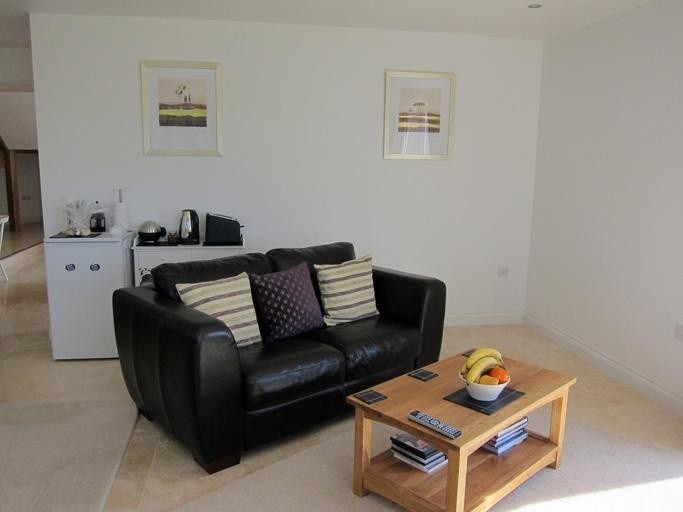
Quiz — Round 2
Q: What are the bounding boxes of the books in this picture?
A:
[480,416,529,456]
[389,429,449,474]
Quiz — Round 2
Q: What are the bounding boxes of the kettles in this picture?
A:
[179,210,200,244]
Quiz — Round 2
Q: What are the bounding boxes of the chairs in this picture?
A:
[0,215,10,281]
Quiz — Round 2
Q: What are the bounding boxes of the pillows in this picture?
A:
[250,262,324,342]
[314,254,380,326]
[176,272,262,348]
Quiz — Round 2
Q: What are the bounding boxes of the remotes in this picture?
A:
[408,410,462,439]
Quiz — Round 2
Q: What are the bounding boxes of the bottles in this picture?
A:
[90,213,105,232]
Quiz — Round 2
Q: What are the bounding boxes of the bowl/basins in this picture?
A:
[458,371,511,402]
[138,232,161,244]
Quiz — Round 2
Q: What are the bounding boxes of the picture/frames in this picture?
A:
[140,59,224,156]
[383,70,457,160]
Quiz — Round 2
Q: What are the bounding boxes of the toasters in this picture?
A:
[205,212,243,243]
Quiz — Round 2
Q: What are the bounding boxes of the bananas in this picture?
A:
[461,347,506,386]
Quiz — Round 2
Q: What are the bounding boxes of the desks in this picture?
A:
[346,347,577,512]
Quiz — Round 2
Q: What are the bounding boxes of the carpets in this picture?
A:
[0,399,139,512]
[168,409,683,512]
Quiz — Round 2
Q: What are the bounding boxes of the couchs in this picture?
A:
[113,242,447,474]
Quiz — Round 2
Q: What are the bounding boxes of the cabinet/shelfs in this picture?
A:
[43,232,136,360]
[131,235,247,287]
